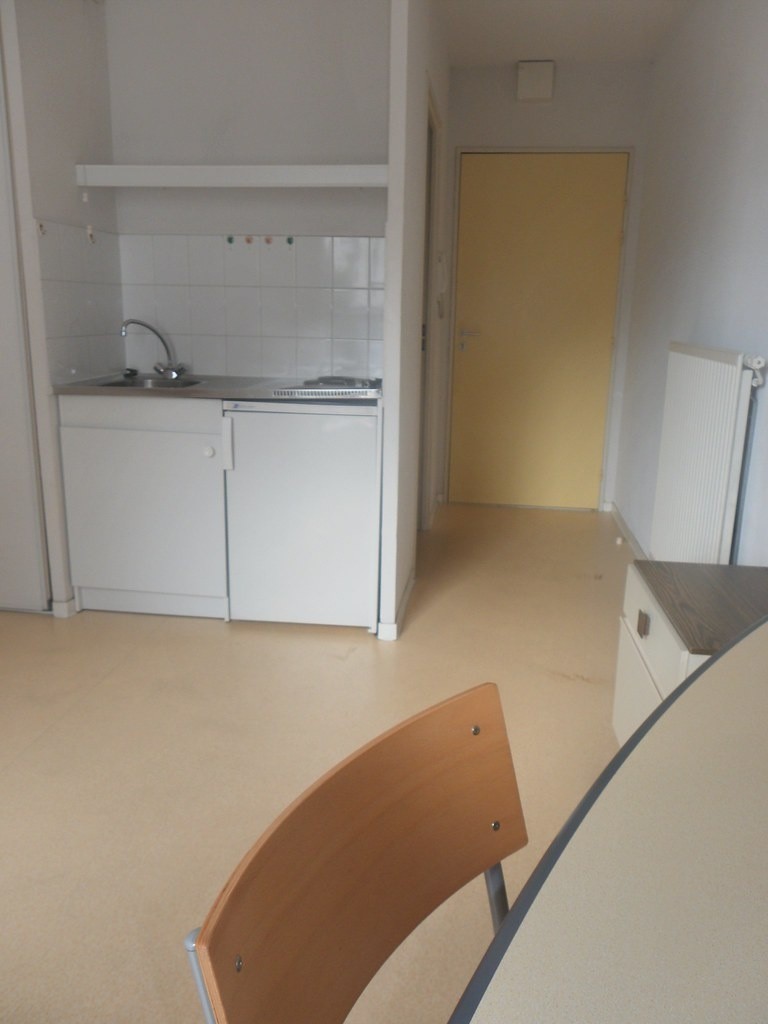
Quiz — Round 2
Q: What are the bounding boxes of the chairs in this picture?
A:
[189,676,528,1024]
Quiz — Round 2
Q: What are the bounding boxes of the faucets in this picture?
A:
[120,318,190,380]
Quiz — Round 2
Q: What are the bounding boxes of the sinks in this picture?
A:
[96,375,207,391]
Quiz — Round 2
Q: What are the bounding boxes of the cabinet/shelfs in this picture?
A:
[59,404,394,636]
[606,557,768,752]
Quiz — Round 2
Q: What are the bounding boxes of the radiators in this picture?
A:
[642,341,768,569]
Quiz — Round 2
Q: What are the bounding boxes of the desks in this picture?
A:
[447,610,768,1024]
